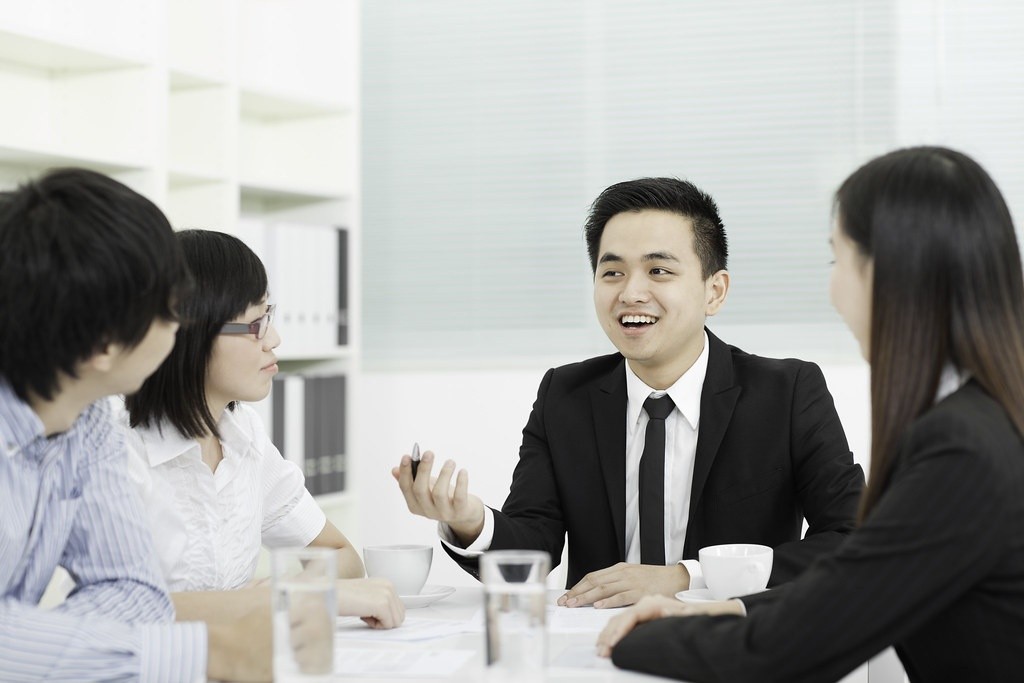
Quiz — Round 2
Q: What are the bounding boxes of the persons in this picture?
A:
[126,226,408,630]
[393,180,866,608]
[597,146,1024,683]
[0,167,273,683]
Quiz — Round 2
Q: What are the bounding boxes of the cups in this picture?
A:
[479,550,550,678]
[270,547,338,682]
[698,544,774,599]
[362,544,433,595]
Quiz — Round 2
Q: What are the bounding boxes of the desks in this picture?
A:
[274,568,874,683]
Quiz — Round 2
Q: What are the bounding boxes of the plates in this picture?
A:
[338,579,455,609]
[675,589,773,607]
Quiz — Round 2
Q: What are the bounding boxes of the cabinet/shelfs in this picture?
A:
[0,41,377,509]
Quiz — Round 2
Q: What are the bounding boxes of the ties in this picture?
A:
[639,394,676,566]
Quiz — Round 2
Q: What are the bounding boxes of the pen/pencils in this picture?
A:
[411,442,421,481]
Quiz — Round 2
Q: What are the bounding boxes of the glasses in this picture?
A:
[219,305,276,339]
